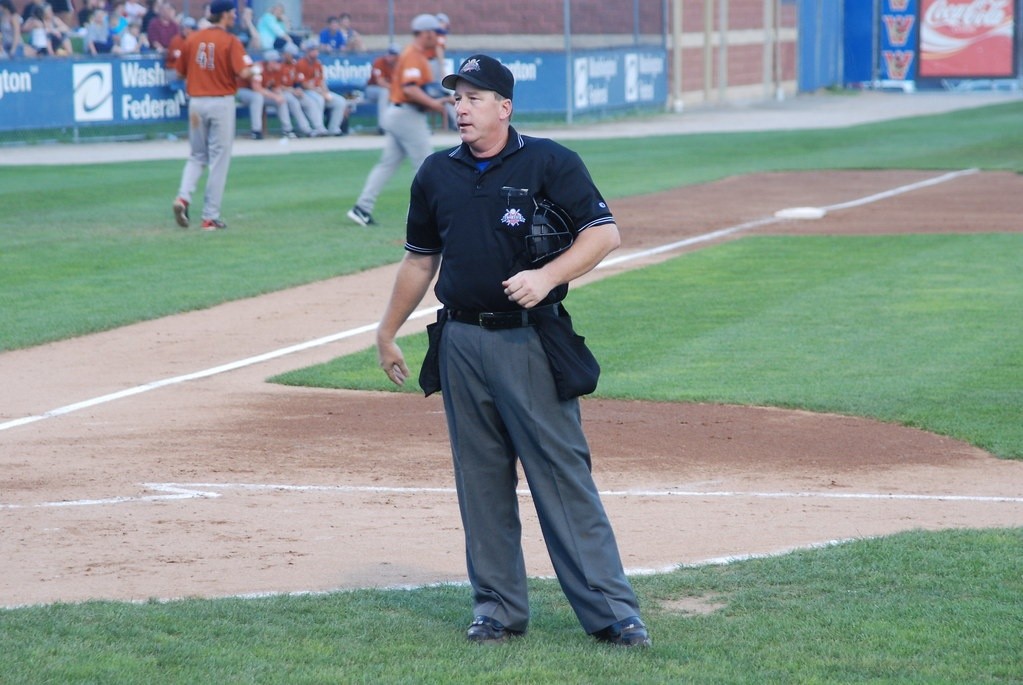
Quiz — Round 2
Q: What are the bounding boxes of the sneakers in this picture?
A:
[201,219,227,231]
[172,197,191,227]
[591,616,653,648]
[467,615,528,643]
[346,204,377,227]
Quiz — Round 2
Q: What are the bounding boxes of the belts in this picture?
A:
[445,308,538,329]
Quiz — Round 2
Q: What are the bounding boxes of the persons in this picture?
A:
[171,0,255,229]
[0,0,451,139]
[347,13,455,228]
[376,55,651,646]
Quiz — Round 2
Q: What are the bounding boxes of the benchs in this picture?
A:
[262,97,447,137]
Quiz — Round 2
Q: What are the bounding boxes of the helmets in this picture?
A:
[494,186,579,304]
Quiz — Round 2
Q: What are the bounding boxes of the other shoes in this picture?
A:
[282,131,297,139]
[250,132,263,139]
[307,128,341,137]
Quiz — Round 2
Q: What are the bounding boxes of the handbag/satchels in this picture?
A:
[528,302,601,402]
[418,310,448,398]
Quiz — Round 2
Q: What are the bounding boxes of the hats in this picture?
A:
[411,13,449,35]
[436,13,450,23]
[210,0,238,13]
[441,55,514,99]
